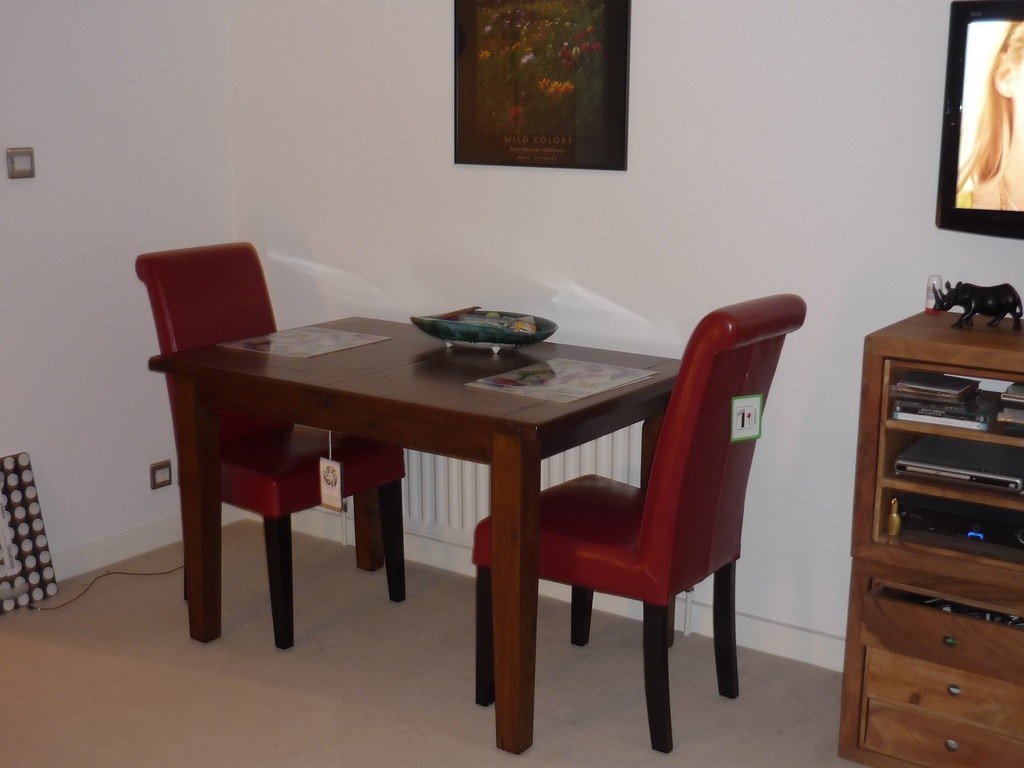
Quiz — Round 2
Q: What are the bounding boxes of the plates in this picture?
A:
[410,311,559,344]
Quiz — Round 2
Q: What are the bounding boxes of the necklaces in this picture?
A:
[1000,179,1006,211]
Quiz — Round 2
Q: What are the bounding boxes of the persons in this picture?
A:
[956,21,1024,211]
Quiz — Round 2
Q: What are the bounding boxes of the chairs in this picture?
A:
[473,292,807,754]
[136,240,408,650]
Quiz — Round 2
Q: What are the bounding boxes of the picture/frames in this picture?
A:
[452,0,630,171]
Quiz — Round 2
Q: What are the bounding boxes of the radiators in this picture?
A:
[345,417,645,550]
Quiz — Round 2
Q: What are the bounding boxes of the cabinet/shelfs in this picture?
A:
[837,309,1024,768]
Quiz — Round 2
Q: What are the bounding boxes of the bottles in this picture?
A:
[887,498,901,536]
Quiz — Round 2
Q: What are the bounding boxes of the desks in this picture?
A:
[148,318,686,705]
